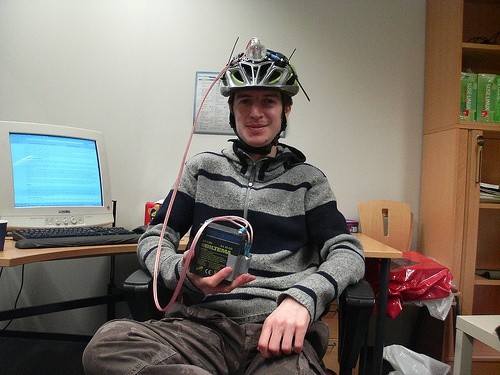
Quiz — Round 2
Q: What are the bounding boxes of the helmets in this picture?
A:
[220,49,299,97]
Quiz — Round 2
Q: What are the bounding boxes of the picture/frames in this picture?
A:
[192,71,285,137]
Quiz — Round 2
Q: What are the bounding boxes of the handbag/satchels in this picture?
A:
[383,345,451,375]
[376,251,454,301]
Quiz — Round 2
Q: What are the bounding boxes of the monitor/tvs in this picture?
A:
[0,121,114,230]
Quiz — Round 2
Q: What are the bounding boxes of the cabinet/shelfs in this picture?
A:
[417,0,500,375]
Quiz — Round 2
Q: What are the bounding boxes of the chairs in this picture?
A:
[123,268,376,375]
[357,200,455,366]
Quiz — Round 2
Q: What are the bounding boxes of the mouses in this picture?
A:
[132,225,146,234]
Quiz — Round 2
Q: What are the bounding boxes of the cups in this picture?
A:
[0,219,8,251]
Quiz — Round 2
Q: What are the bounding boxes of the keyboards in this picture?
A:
[12,227,128,241]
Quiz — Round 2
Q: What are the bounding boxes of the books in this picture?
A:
[480,182,500,203]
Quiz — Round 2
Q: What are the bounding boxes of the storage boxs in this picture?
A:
[476,73,500,122]
[459,71,477,121]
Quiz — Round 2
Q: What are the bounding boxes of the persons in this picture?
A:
[83,37,365,375]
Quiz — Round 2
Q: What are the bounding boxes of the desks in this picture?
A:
[0,233,404,375]
[453,314,500,375]
